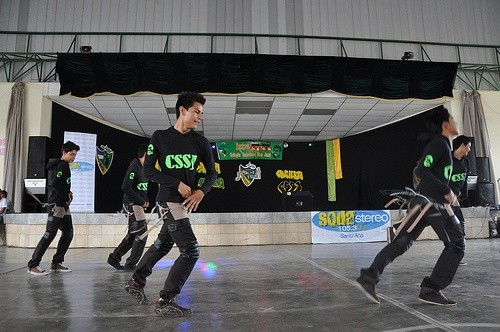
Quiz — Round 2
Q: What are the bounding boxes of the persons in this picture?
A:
[388,135,472,266]
[124,92,217,317]
[107,144,150,271]
[27,141,81,276]
[355,107,467,306]
[0,189,15,247]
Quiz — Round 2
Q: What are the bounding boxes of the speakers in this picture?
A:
[478,182,494,206]
[476,157,491,183]
[27,136,58,179]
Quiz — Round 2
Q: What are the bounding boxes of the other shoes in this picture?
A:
[125,267,136,272]
[107,259,125,270]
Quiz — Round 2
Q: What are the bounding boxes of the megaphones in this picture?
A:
[80,46,92,53]
[401,52,414,60]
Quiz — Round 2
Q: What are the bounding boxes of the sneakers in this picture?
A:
[387,226,395,244]
[418,287,457,306]
[49,262,71,272]
[355,277,381,304]
[27,266,48,275]
[156,300,192,316]
[124,278,150,305]
